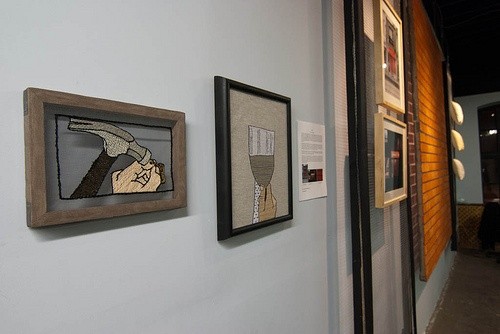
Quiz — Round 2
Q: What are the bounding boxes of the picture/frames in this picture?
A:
[374,111,408,209]
[372,0,407,114]
[22,86,188,228]
[213,74,295,241]
